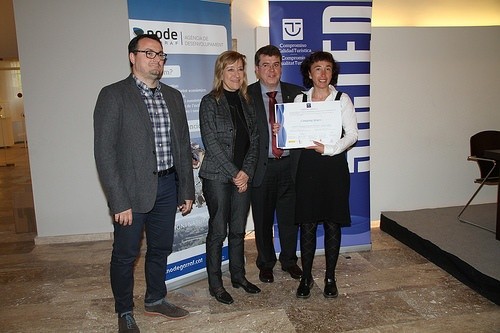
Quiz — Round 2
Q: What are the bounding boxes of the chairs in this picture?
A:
[456,130,500,233]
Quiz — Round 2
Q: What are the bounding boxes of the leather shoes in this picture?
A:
[323,276,338,297]
[296,274,314,299]
[210,286,234,305]
[282,264,303,279]
[232,277,261,294]
[259,269,274,283]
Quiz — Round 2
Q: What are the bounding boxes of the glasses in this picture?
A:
[132,50,167,60]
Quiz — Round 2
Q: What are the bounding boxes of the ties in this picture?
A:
[266,91,284,159]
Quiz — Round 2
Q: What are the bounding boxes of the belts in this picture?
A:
[158,167,174,176]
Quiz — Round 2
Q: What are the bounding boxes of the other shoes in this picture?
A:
[118,312,140,333]
[145,300,189,319]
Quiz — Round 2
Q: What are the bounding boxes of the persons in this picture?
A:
[94,34,195,333]
[273,51,358,298]
[247,45,307,282]
[198,51,261,304]
